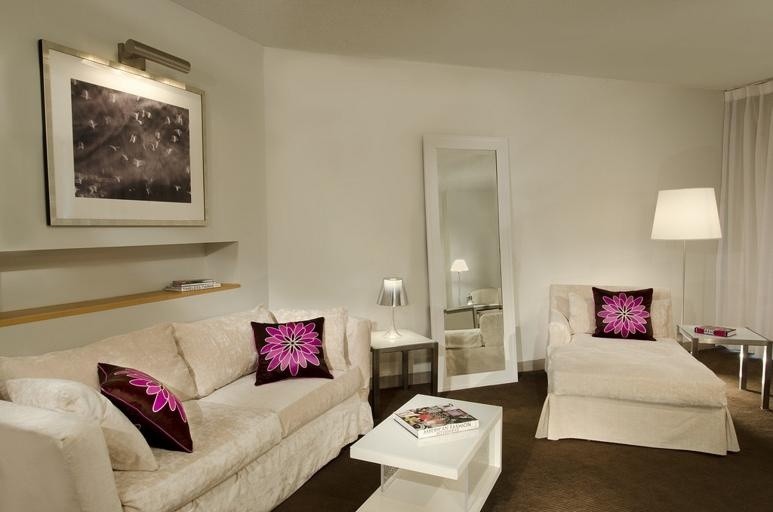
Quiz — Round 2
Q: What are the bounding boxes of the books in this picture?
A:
[393,403,479,439]
[695,326,736,337]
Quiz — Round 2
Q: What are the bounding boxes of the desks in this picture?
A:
[445,303,503,329]
[674,324,773,410]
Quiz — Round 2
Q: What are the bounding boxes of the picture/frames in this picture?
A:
[38,39,210,227]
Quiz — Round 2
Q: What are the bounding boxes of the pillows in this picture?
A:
[97,362,193,453]
[6,378,157,472]
[251,317,334,386]
[0,325,197,420]
[172,304,275,397]
[568,292,669,339]
[271,307,348,371]
[592,286,656,340]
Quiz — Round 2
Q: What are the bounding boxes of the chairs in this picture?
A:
[472,288,501,328]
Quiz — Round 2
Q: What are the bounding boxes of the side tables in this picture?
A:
[370,329,438,418]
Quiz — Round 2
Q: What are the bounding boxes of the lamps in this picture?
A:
[377,277,408,338]
[651,187,722,325]
[450,259,469,306]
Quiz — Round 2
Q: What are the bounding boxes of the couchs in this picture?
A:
[444,312,505,377]
[0,315,374,512]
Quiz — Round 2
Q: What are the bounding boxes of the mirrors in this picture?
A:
[422,132,518,393]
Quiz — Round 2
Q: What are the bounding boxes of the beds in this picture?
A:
[535,283,740,456]
